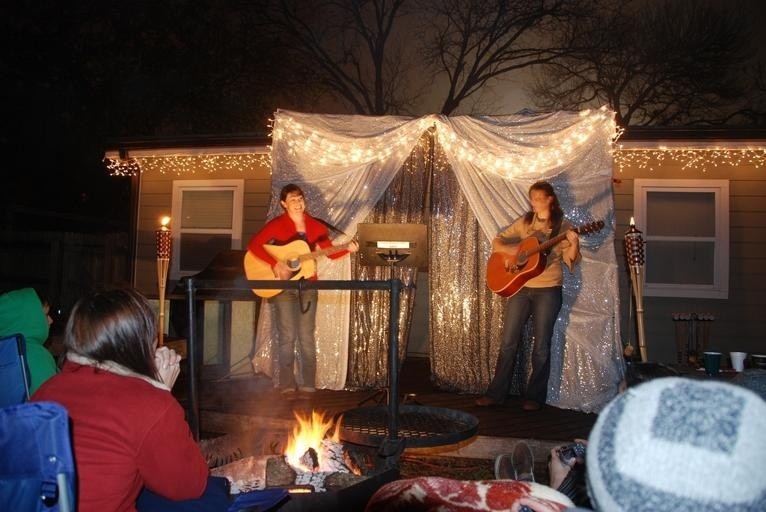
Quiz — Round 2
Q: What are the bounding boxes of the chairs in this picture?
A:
[0,334,35,403]
[0,400,288,512]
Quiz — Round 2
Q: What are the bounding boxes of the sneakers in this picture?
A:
[495,453,514,480]
[512,440,535,482]
[283,391,313,400]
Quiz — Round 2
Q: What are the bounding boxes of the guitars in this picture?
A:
[487,222,605,296]
[244,228,358,299]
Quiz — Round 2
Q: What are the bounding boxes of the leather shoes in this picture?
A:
[523,400,540,410]
[476,396,496,406]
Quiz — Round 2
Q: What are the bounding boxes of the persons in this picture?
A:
[26,286,209,512]
[0,285,63,399]
[492,348,766,512]
[246,183,360,401]
[473,180,583,413]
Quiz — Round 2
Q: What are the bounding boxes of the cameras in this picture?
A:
[558,441,586,465]
